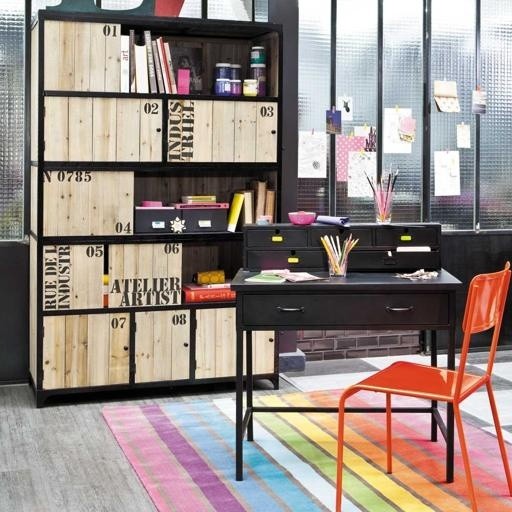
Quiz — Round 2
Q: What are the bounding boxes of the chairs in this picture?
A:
[337,260,512,512]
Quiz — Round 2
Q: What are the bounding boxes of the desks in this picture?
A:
[232,266,462,481]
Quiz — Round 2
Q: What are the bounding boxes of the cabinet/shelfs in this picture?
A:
[30,11,280,409]
[244,223,440,267]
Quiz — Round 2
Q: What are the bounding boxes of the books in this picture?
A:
[170,181,275,232]
[183,281,235,303]
[122,28,203,95]
[243,273,286,284]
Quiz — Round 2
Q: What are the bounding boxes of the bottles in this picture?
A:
[242,46,267,98]
[215,63,242,96]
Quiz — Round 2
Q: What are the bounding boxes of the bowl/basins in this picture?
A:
[287,211,317,225]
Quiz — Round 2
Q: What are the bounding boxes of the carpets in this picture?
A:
[100,388,512,512]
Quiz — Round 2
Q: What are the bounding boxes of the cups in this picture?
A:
[329,260,348,278]
[374,191,394,225]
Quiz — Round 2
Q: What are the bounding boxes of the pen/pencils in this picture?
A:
[363,162,399,224]
[320,233,360,277]
